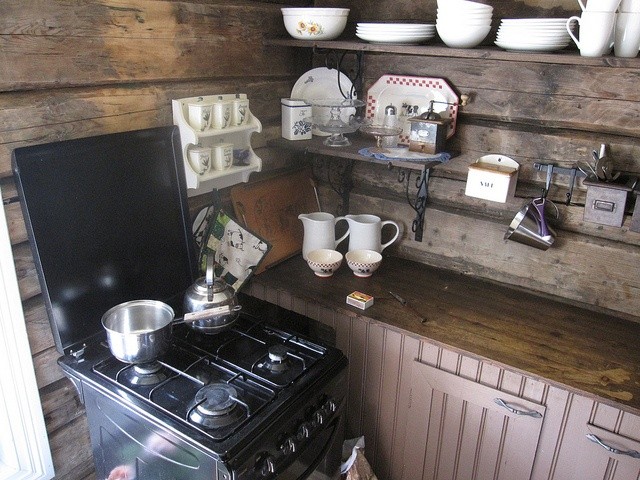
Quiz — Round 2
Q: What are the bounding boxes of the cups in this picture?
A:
[577,0,621,12]
[213,95,232,130]
[232,93,249,127]
[619,0,640,12]
[188,96,212,131]
[616,13,640,57]
[565,11,614,57]
[212,139,232,171]
[190,144,213,174]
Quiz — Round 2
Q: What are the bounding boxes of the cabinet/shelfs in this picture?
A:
[412,362,571,480]
[171,94,263,197]
[270,126,462,243]
[558,395,638,479]
[253,283,426,480]
[262,36,640,70]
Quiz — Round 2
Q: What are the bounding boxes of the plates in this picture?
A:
[353,22,435,44]
[291,68,357,137]
[493,18,573,50]
[364,74,458,146]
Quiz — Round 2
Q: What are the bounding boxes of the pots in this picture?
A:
[99,300,240,365]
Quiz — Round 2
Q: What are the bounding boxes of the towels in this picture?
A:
[197,204,273,295]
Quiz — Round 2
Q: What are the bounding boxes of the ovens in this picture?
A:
[230,380,356,480]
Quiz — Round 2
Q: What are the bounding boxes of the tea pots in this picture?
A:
[297,212,349,261]
[344,214,399,254]
[183,254,242,332]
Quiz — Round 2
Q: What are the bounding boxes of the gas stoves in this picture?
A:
[88,313,349,457]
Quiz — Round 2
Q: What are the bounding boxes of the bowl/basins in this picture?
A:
[433,1,493,47]
[305,248,343,277]
[279,8,350,41]
[345,248,383,277]
